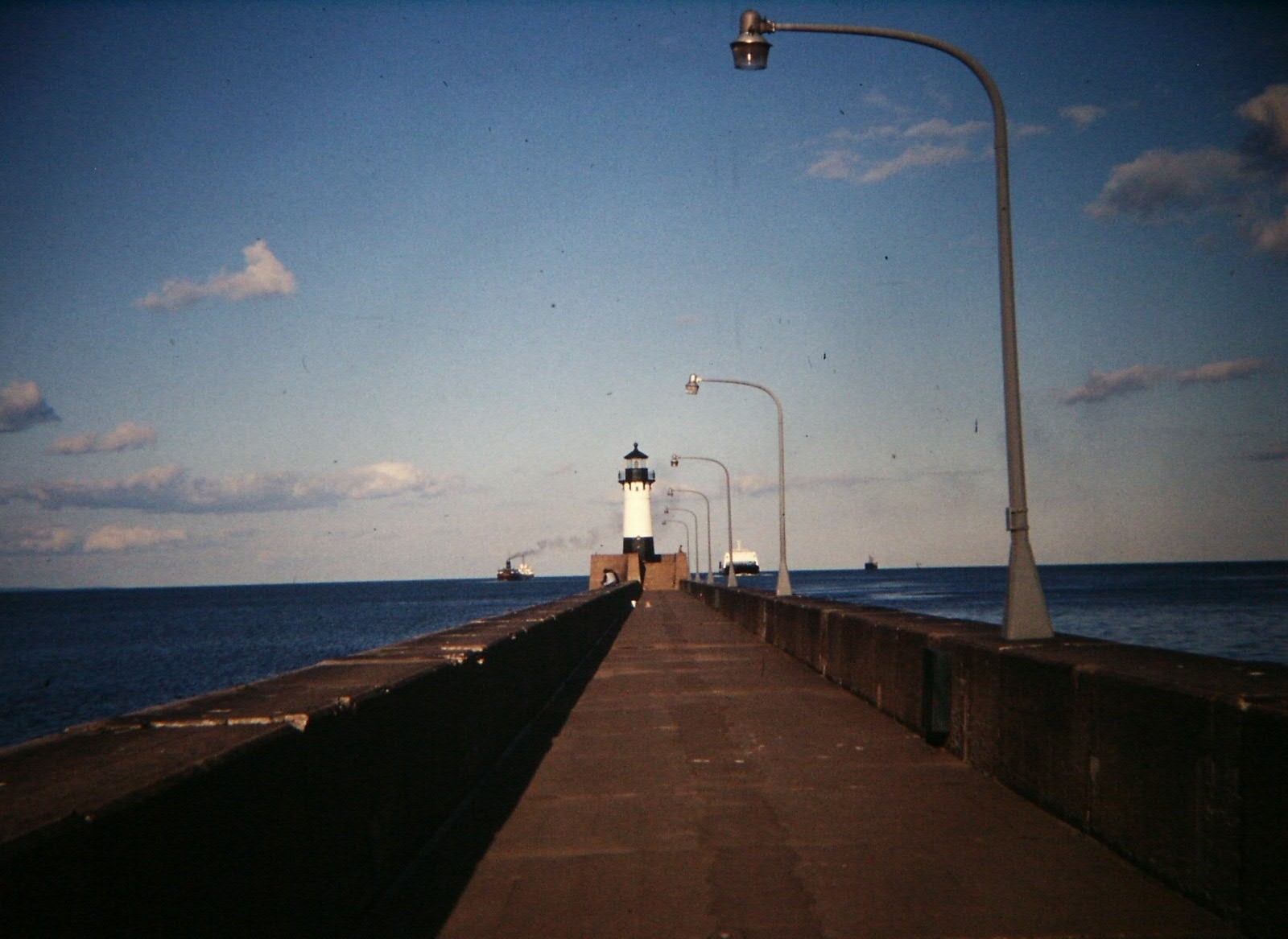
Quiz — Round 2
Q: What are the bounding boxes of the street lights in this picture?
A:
[668,487,716,585]
[670,454,740,587]
[727,9,1054,640]
[662,519,692,581]
[684,375,793,597]
[664,506,701,583]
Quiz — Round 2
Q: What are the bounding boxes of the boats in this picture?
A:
[719,540,760,576]
[864,555,879,569]
[497,559,535,581]
